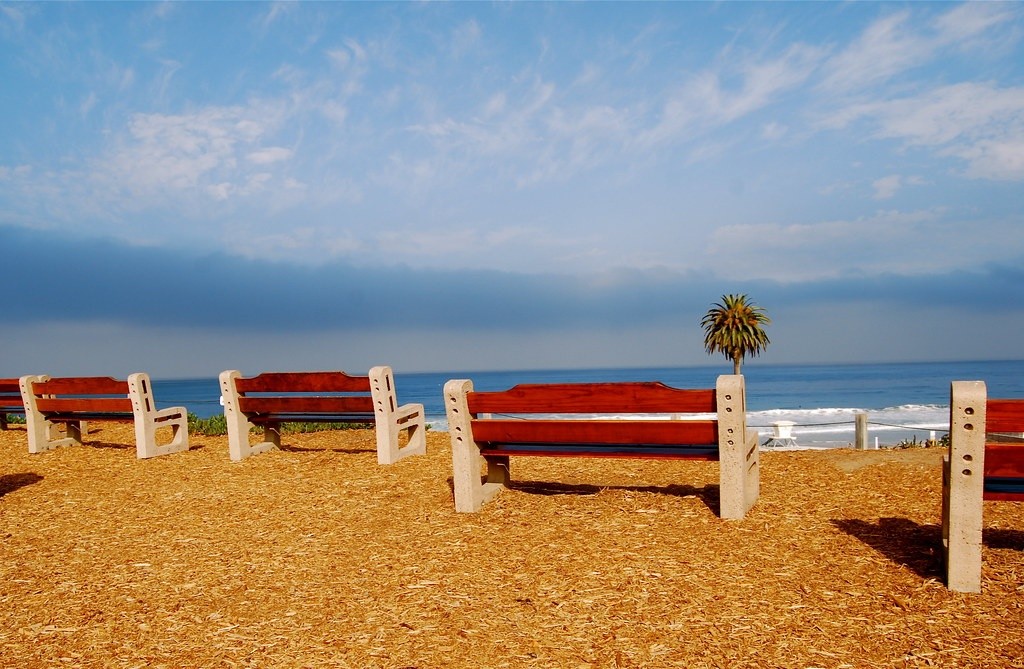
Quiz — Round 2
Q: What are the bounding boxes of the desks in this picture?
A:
[769,437,797,446]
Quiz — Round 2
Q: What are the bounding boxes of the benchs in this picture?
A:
[444,375,760,521]
[0,373,190,460]
[941,380,1024,594]
[218,366,426,463]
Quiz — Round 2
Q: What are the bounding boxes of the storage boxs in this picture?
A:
[773,421,794,437]
